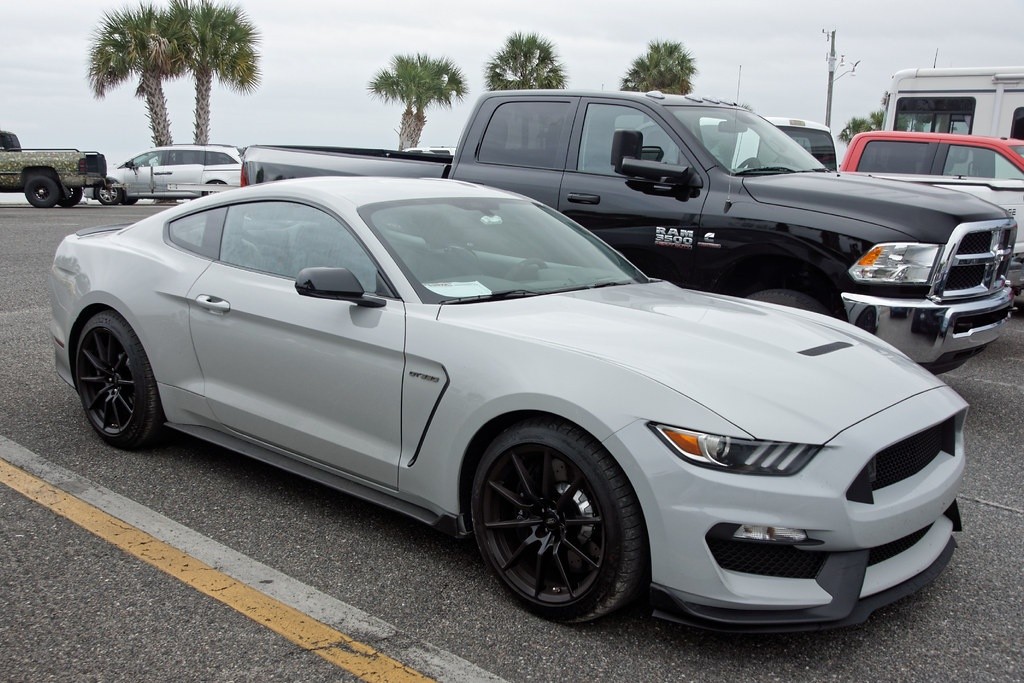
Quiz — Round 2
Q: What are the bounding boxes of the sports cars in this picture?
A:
[48,174,972,630]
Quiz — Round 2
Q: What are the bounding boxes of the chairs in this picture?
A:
[224,209,264,269]
[422,217,476,281]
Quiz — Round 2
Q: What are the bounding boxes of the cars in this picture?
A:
[80,143,244,205]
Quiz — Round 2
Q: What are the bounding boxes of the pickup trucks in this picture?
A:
[236,89,1017,380]
[632,66,1024,295]
[0,130,107,209]
